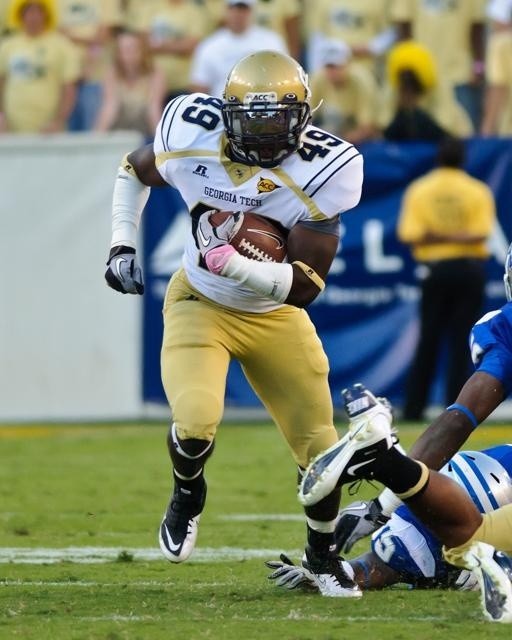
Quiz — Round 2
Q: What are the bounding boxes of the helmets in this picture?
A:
[222,50,309,167]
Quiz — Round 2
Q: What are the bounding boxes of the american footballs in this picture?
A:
[209,209,293,267]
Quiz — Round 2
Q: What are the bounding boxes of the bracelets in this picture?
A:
[378,486,405,519]
[219,252,293,305]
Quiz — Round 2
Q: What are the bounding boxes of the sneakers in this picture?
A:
[158,478,207,562]
[295,416,392,507]
[464,541,511,623]
[342,385,393,415]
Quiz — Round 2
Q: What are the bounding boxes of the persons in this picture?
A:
[265,384,512,594]
[334,241,512,554]
[0,0,512,143]
[296,410,512,625]
[395,138,496,422]
[104,49,364,598]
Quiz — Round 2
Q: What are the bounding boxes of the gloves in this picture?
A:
[267,554,318,592]
[106,244,144,295]
[330,499,392,555]
[197,209,239,259]
